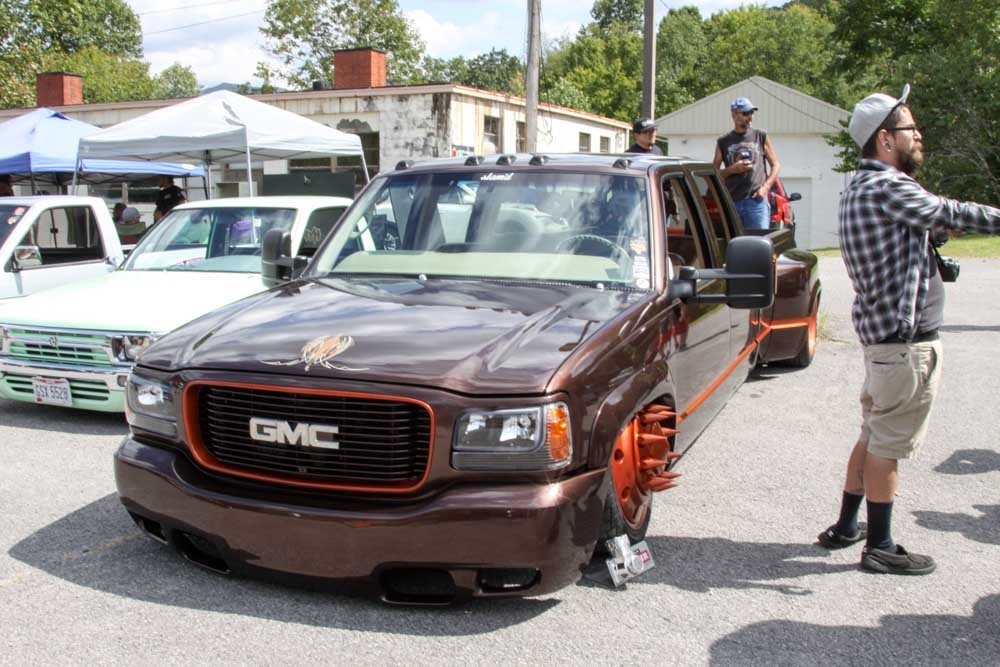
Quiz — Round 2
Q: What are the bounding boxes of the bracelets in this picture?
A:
[674,213,679,215]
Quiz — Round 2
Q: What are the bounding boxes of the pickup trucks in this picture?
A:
[1,195,207,303]
[669,176,802,241]
[111,150,819,609]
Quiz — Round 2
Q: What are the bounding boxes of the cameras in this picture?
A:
[937,256,960,282]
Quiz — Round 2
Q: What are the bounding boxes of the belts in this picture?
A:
[877,330,940,344]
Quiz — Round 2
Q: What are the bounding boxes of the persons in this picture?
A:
[0,175,14,196]
[624,118,680,227]
[712,97,780,230]
[153,176,187,224]
[123,207,140,223]
[819,84,1000,576]
[113,204,127,223]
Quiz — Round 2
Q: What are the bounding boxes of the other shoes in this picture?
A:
[817,521,868,549]
[861,544,936,575]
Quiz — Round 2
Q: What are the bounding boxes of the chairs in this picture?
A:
[603,194,654,239]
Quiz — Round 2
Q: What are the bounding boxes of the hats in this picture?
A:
[155,185,185,211]
[848,83,911,149]
[633,118,659,132]
[122,206,145,222]
[730,97,758,112]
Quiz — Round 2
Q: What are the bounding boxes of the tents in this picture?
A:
[0,107,207,204]
[70,90,378,246]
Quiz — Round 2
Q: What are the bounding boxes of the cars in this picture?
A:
[371,176,570,255]
[1,195,379,415]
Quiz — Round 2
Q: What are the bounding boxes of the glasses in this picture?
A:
[884,123,918,137]
[735,110,753,116]
